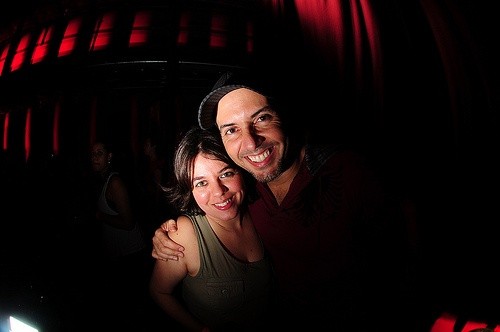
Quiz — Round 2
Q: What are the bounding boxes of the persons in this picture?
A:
[149,125,281,332]
[143,135,165,191]
[151,62,420,332]
[90,141,141,266]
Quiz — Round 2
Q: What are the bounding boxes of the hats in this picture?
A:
[196,67,270,136]
